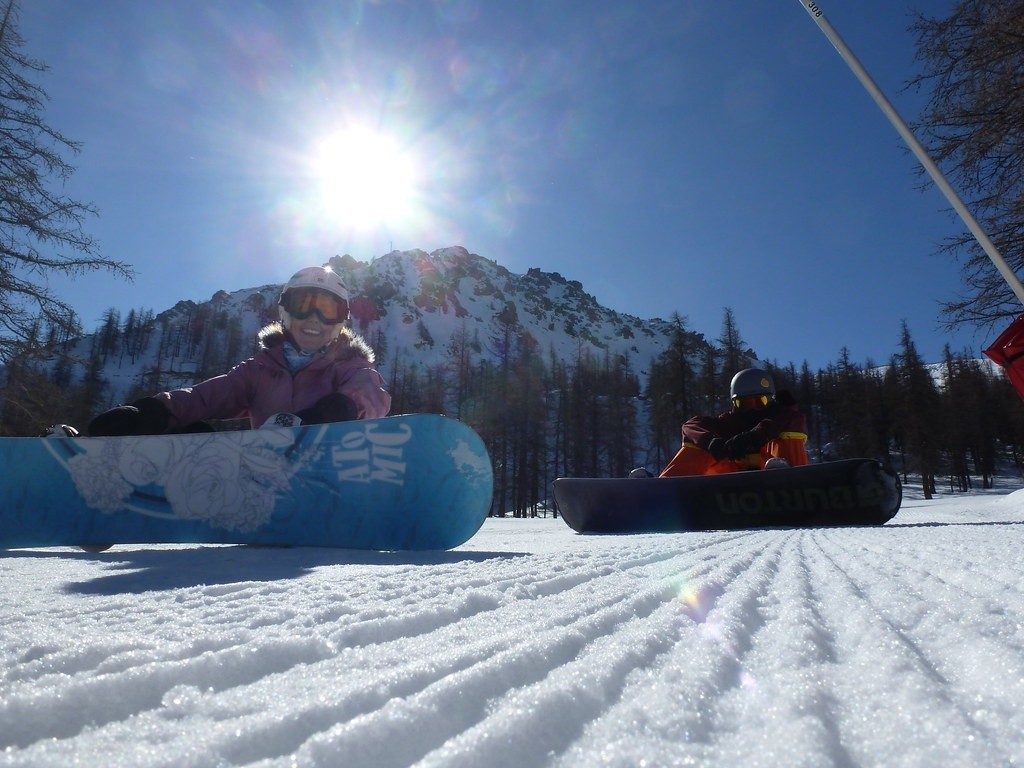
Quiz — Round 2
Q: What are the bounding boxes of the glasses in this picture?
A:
[735,395,768,408]
[282,288,351,324]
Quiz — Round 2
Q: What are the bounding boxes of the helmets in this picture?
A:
[730,368,775,401]
[282,266,349,308]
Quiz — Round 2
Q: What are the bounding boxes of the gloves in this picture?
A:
[710,439,736,461]
[725,433,755,462]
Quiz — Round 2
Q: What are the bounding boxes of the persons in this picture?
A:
[36,266,391,436]
[628,368,809,479]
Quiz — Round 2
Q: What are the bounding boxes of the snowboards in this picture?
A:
[550,455,903,536]
[2,409,496,553]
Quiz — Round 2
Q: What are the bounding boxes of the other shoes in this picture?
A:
[764,457,789,469]
[629,467,654,478]
